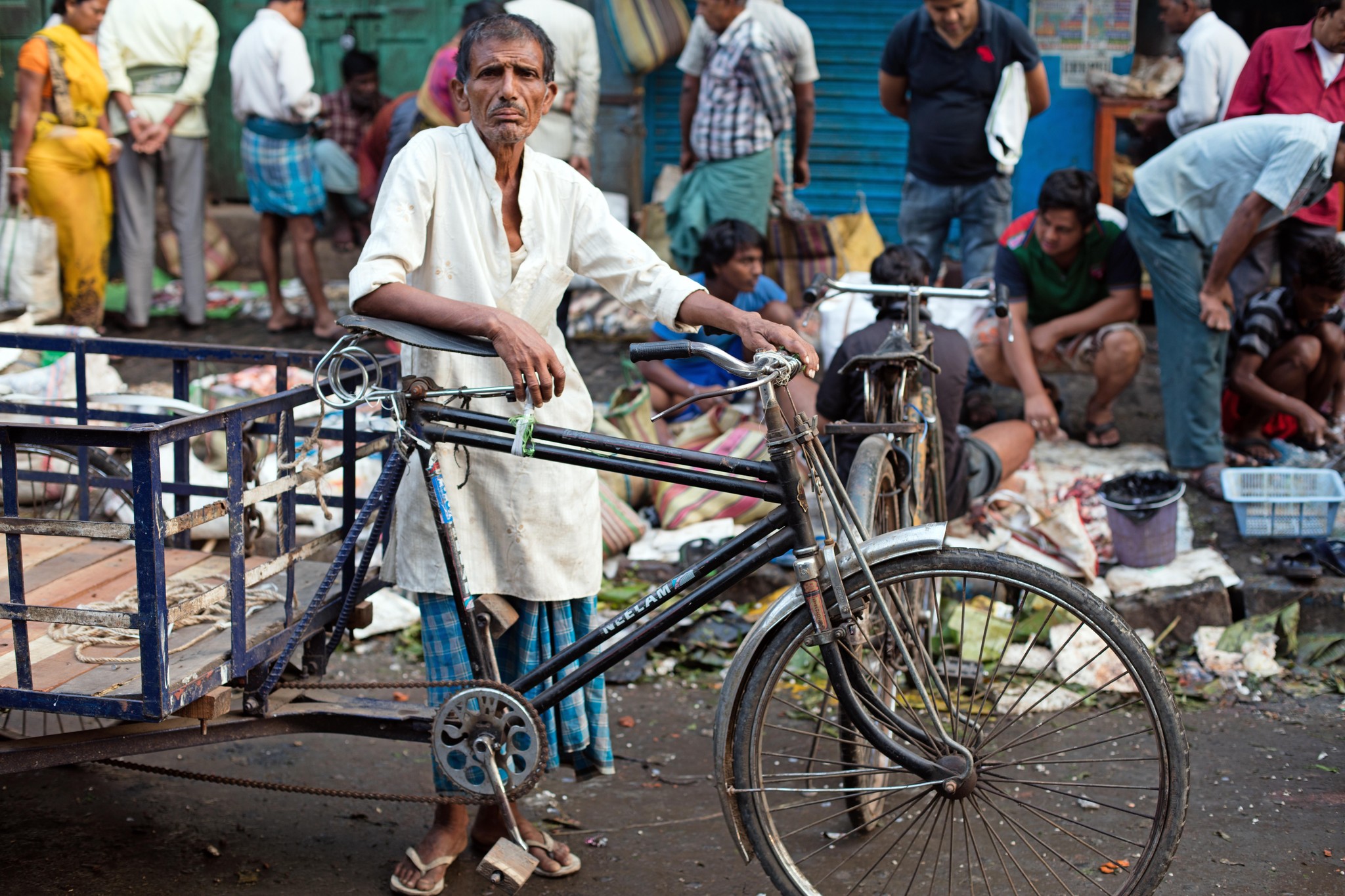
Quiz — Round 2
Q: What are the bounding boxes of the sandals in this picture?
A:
[1186,462,1230,502]
[1223,449,1258,468]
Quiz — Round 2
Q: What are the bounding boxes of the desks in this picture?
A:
[1092,86,1181,301]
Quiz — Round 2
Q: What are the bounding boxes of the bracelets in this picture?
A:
[124,109,140,122]
[5,166,31,177]
[163,118,175,128]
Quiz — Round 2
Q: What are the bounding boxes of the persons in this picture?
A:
[628,218,797,447]
[306,50,392,252]
[662,1,822,273]
[96,1,220,332]
[228,0,372,345]
[1130,0,1252,148]
[876,1,1052,292]
[406,0,505,142]
[812,245,1037,509]
[970,168,1146,449]
[1224,0,1345,286]
[344,14,825,896]
[498,0,604,356]
[6,0,127,364]
[1219,231,1344,464]
[1124,114,1345,501]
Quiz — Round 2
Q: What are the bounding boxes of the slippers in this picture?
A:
[1227,433,1283,463]
[1307,536,1345,576]
[1266,549,1323,578]
[314,324,371,341]
[271,315,314,331]
[389,846,458,896]
[1083,420,1122,447]
[470,826,581,877]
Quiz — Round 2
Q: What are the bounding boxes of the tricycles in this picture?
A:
[0,314,1191,896]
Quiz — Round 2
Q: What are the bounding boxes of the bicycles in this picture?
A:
[801,277,1016,832]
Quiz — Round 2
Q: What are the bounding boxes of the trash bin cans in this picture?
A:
[1097,470,1188,568]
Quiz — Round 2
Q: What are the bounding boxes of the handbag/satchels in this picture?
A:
[985,63,1035,176]
[0,196,63,324]
